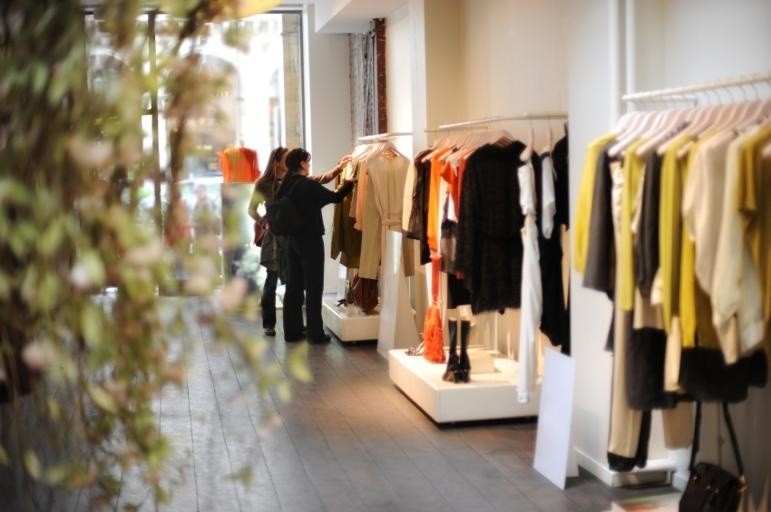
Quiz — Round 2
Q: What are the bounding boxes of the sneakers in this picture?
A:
[264,327,275,335]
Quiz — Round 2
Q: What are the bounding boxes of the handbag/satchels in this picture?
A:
[254,214,268,246]
[353,275,379,313]
[679,399,746,512]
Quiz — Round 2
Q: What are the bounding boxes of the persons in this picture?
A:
[192,183,221,279]
[249,146,352,336]
[276,149,354,344]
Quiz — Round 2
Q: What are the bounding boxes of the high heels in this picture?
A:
[406,339,425,356]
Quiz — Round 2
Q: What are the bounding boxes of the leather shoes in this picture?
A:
[309,333,330,344]
[285,327,307,340]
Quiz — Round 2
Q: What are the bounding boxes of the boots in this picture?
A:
[443,318,459,384]
[460,320,470,383]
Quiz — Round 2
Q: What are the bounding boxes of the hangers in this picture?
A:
[520,112,535,161]
[351,134,403,162]
[422,117,515,163]
[545,113,552,149]
[605,71,771,158]
